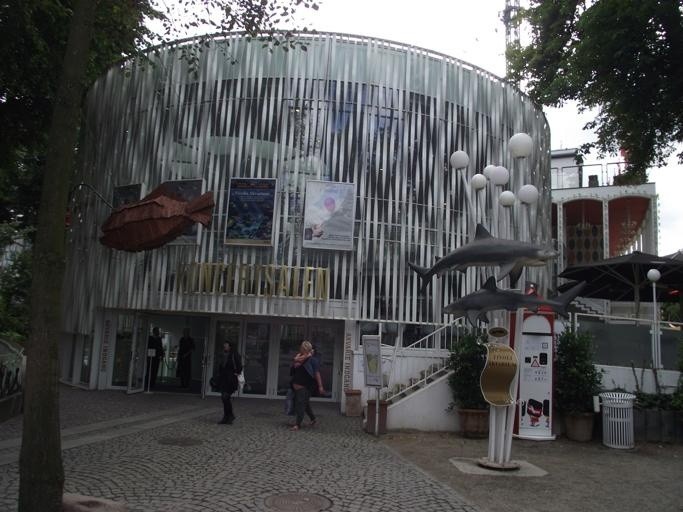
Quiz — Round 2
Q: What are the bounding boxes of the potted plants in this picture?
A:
[552,323,606,442]
[441,324,490,439]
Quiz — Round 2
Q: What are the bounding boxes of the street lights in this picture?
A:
[452,130,537,471]
[645,268,665,372]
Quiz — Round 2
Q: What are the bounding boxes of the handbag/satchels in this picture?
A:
[237,370,245,392]
[284,387,296,416]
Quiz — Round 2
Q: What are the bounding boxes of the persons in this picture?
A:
[145,327,162,389]
[176,328,194,388]
[214,341,242,424]
[289,341,325,430]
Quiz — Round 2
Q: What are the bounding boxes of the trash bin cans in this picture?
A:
[599,392,637,449]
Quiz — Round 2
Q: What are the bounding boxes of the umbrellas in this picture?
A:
[557,252,683,318]
